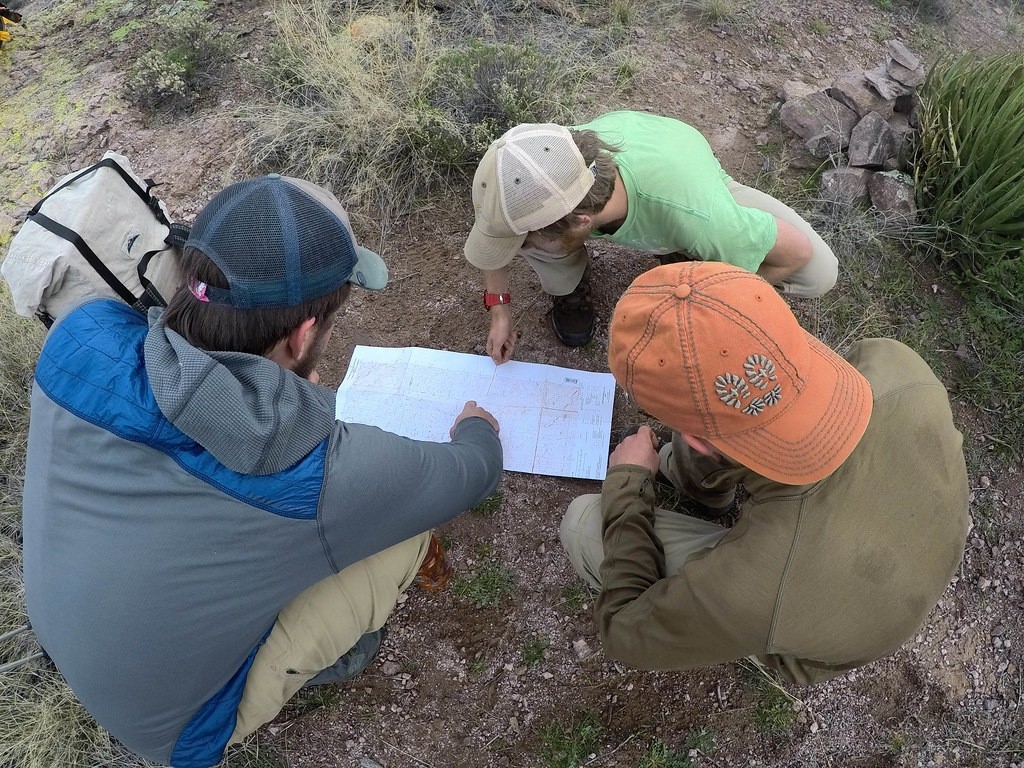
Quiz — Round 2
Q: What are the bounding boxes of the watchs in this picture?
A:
[483,289,510,312]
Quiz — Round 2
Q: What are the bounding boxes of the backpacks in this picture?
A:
[1,150,182,330]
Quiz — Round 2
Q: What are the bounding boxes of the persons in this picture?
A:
[23,174,502,768]
[464,110,838,365]
[558,263,969,686]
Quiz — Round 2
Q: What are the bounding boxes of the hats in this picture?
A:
[464,124,597,269]
[185,173,388,309]
[608,261,873,485]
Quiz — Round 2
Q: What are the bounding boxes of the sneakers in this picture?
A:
[545,260,600,347]
[618,425,737,515]
[303,631,381,686]
